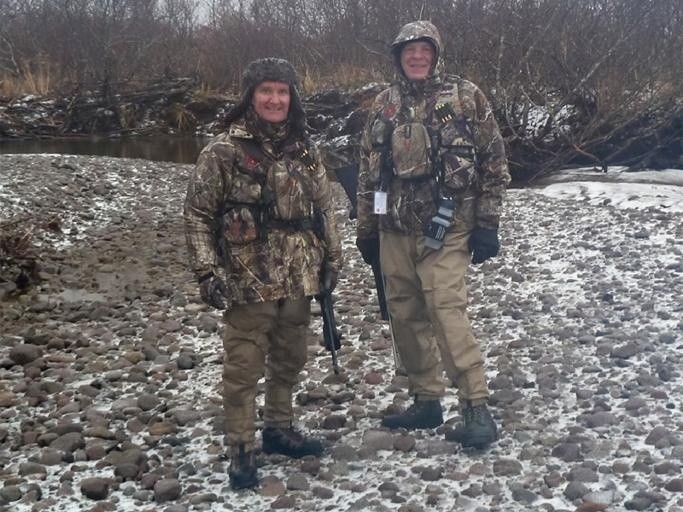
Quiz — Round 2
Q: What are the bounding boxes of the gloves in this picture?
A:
[353,235,378,266]
[466,225,500,266]
[196,270,230,311]
[314,267,340,301]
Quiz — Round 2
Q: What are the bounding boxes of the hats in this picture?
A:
[216,58,307,132]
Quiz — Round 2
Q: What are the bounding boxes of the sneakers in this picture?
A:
[443,402,498,445]
[260,424,326,458]
[380,398,444,430]
[225,451,256,489]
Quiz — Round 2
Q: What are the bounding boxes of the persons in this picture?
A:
[180,56,345,491]
[352,19,510,450]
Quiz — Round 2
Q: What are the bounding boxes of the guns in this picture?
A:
[313,200,342,375]
[335,163,392,322]
